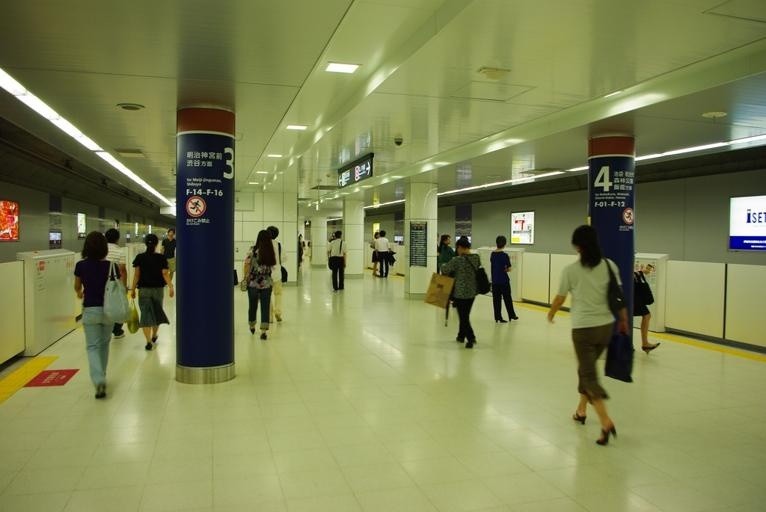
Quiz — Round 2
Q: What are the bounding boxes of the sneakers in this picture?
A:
[259,333,267,340]
[93,383,106,400]
[112,329,125,339]
[144,337,158,350]
[274,312,282,322]
[332,287,343,291]
[455,334,476,349]
[371,273,387,277]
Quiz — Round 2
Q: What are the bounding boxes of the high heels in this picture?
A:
[595,424,618,447]
[249,328,255,335]
[640,343,660,354]
[495,318,507,323]
[508,316,518,322]
[572,412,586,426]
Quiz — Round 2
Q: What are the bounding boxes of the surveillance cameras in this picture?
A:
[394,138,403,146]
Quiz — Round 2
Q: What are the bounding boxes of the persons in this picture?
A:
[375,231,391,277]
[160,228,176,286]
[73,230,120,399]
[370,233,379,277]
[437,233,482,348]
[490,236,519,323]
[132,233,173,350]
[103,229,128,338]
[244,230,275,339]
[326,231,346,290]
[267,226,283,323]
[547,224,627,444]
[633,268,660,354]
[298,234,304,271]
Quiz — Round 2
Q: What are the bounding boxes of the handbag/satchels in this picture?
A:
[474,268,490,294]
[604,332,635,384]
[423,272,455,309]
[239,279,248,292]
[634,271,654,306]
[103,260,129,324]
[606,273,624,311]
[280,266,287,283]
[327,256,346,270]
